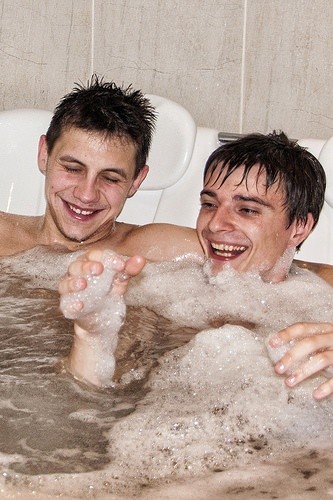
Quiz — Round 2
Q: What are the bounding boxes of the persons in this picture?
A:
[0,72,333,284]
[57,131,333,400]
